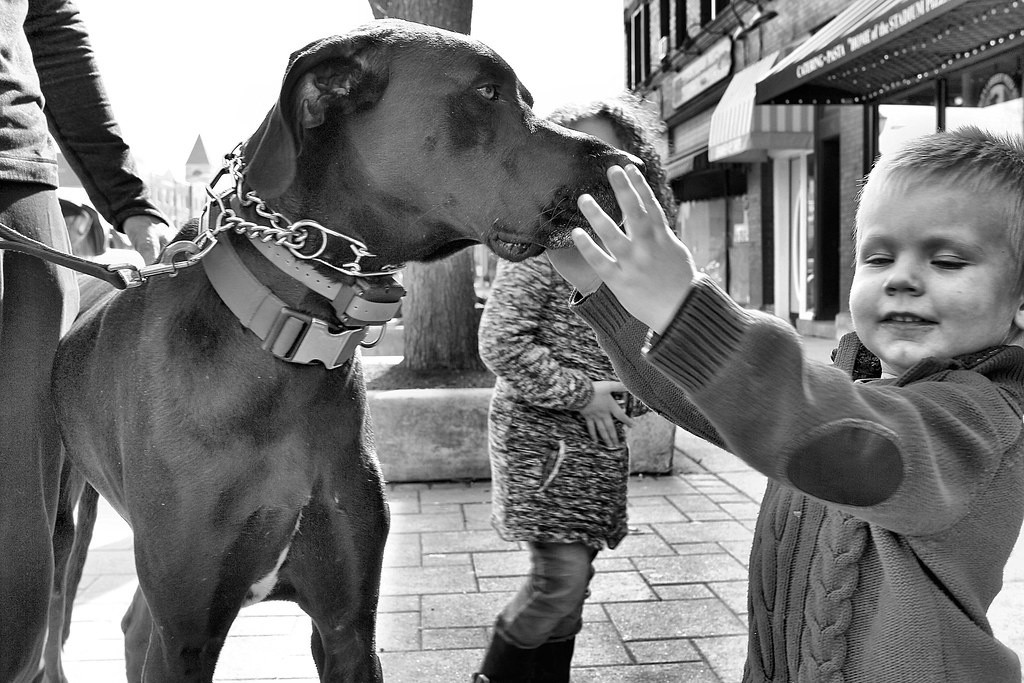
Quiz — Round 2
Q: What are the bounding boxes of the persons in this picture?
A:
[0,0,179,683]
[472,95,679,683]
[545,126,1024,683]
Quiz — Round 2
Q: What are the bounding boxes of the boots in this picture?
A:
[473,628,575,683]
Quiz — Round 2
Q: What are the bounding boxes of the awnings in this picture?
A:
[708,47,814,162]
[659,146,748,185]
[754,0,1023,106]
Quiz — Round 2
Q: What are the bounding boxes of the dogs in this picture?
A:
[49,17,649,683]
